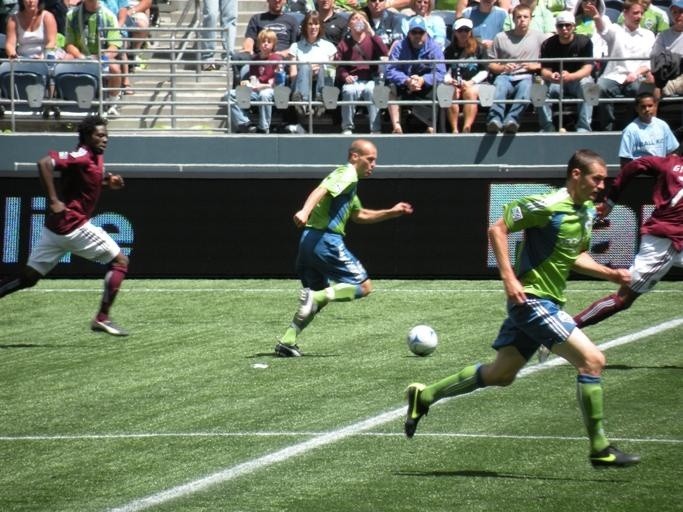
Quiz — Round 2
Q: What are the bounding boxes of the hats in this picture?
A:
[407,15,473,35]
[669,0,683,12]
[555,10,576,25]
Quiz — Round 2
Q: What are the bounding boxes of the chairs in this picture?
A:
[52,58,109,121]
[0,58,49,119]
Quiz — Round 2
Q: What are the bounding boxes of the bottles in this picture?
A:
[274,64,284,87]
[455,67,462,87]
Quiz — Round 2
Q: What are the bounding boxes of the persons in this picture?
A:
[618,92,680,171]
[404,148,644,469]
[271,139,413,359]
[0,0,162,118]
[538,151,682,363]
[223,1,682,131]
[0,118,130,338]
[201,0,238,71]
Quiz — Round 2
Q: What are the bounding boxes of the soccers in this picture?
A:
[407,325,437,355]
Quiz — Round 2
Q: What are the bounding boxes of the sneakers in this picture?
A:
[404,382,429,439]
[589,445,644,469]
[485,120,520,133]
[90,317,129,336]
[274,340,304,357]
[108,105,120,115]
[202,63,216,70]
[293,286,318,327]
[538,344,551,364]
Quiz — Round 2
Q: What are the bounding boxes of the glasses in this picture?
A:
[555,23,575,31]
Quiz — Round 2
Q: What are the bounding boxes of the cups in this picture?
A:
[43,49,57,70]
[100,55,109,72]
